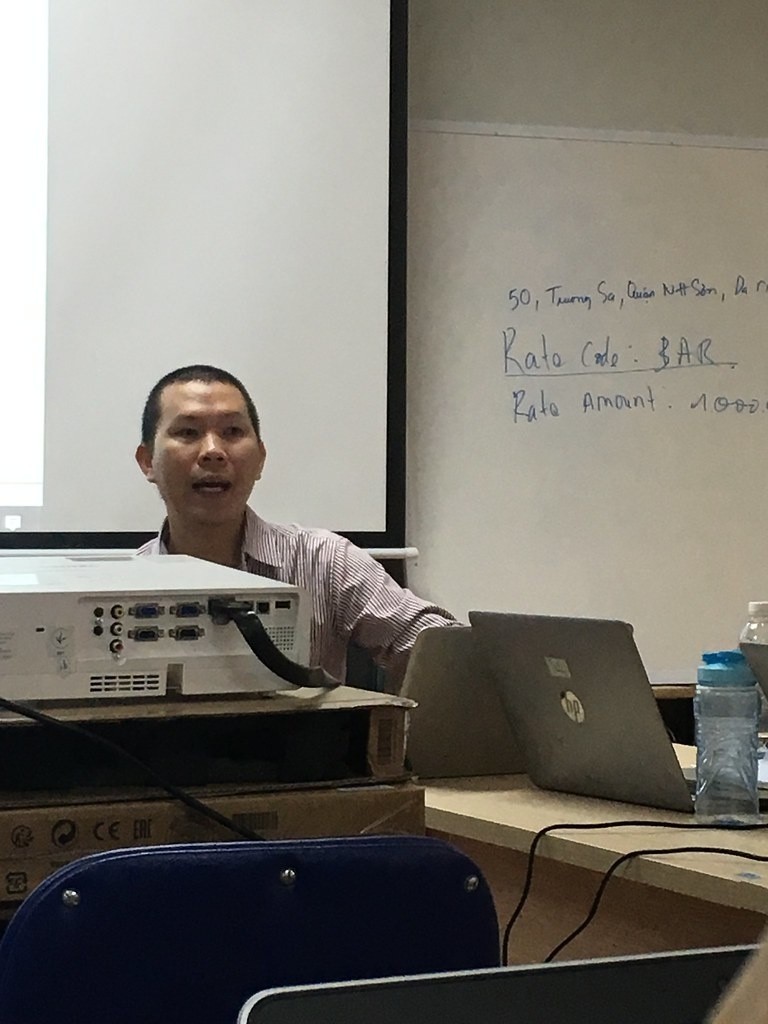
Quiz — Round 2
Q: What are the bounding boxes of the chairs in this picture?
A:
[0,833,500,1024]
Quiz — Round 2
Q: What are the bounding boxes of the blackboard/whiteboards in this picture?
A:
[408,121,768,702]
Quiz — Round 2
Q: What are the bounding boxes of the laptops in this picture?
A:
[399,624,526,782]
[468,611,768,815]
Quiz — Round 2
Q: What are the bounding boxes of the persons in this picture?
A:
[135,365,472,686]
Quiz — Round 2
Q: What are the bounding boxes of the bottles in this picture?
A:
[692,651,759,816]
[739,601,768,644]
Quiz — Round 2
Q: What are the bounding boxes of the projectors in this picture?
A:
[0,548,312,700]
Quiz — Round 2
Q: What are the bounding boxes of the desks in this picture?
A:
[418,739,768,966]
